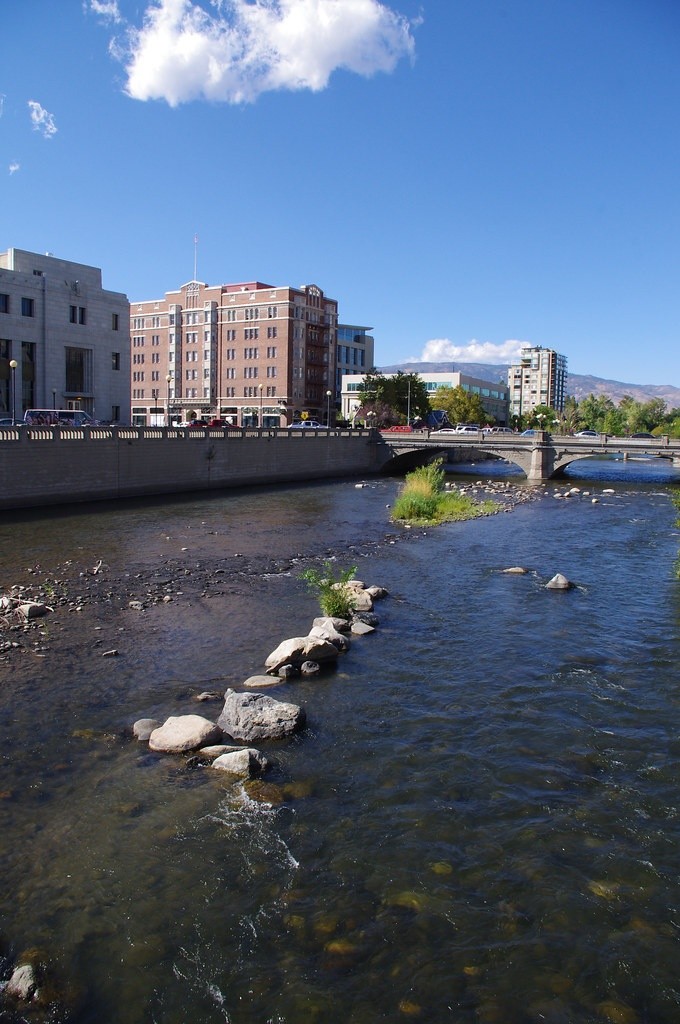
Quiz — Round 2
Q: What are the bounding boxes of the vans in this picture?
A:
[23,409,98,428]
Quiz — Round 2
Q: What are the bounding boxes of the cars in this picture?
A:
[456,424,492,436]
[575,431,599,438]
[186,419,208,428]
[521,430,534,437]
[491,427,514,434]
[430,427,456,433]
[631,432,655,439]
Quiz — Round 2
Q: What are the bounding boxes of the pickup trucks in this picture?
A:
[288,422,324,429]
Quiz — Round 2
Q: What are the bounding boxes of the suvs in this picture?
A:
[380,426,413,432]
[209,419,238,428]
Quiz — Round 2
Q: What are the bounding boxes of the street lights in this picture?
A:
[327,391,331,428]
[167,375,171,427]
[51,388,57,410]
[10,359,17,426]
[259,383,264,428]
